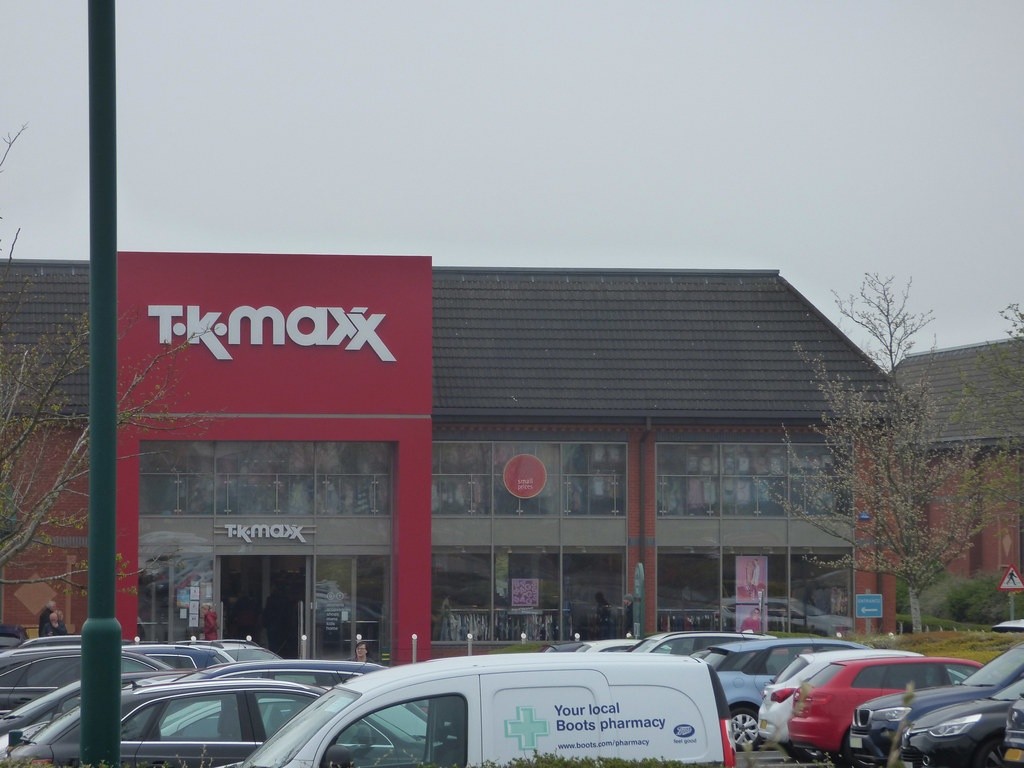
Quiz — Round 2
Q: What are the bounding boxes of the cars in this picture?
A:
[0,621,459,768]
[897,674,1024,768]
[702,637,894,753]
[720,594,856,642]
[1002,692,1024,766]
[788,655,999,768]
[756,649,969,765]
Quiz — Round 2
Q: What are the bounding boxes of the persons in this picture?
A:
[202,603,217,640]
[596,591,611,640]
[741,609,762,633]
[623,594,634,639]
[746,559,760,598]
[38,600,67,637]
[353,642,374,664]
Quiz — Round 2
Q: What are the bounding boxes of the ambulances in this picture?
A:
[209,649,737,768]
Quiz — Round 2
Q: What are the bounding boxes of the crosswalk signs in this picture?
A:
[996,564,1024,592]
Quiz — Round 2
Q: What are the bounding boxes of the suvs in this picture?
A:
[538,638,672,654]
[848,639,1024,768]
[622,630,813,658]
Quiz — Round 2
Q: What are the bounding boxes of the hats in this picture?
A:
[623,593,633,602]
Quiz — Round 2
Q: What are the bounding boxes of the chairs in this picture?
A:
[218,706,240,741]
[137,709,160,741]
